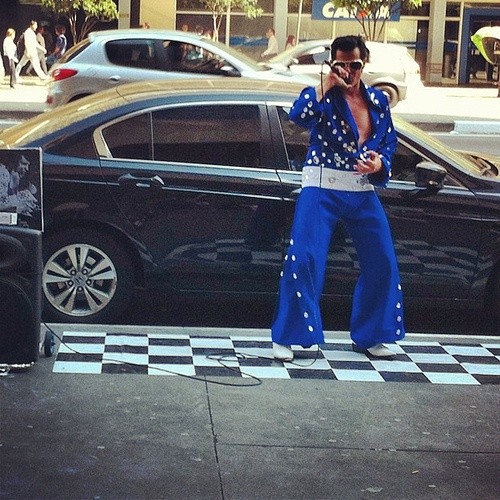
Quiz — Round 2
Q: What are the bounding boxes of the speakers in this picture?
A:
[0,226,43,366]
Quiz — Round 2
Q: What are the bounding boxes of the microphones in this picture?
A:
[325,61,354,85]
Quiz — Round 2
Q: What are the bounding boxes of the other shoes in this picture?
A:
[10,86,18,90]
[25,72,31,77]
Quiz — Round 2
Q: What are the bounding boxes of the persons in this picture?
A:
[203,28,212,39]
[195,24,203,35]
[261,28,277,61]
[1,20,71,87]
[270,33,407,362]
[285,35,296,51]
[181,24,190,32]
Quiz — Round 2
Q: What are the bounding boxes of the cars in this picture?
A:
[1,83,499,337]
[46,28,323,112]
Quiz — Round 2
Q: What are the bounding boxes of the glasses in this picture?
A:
[329,59,365,70]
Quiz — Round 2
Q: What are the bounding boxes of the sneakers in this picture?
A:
[272,341,293,362]
[352,340,398,360]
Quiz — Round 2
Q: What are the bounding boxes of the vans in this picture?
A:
[259,38,422,108]
